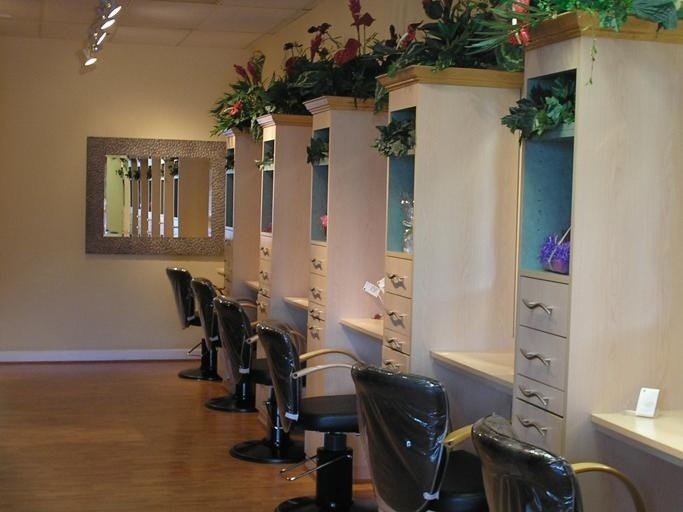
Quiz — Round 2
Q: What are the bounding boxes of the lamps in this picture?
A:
[79,0,122,68]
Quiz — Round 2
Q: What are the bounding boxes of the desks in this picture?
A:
[590,405,682,470]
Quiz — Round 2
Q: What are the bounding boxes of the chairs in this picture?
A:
[231,321,307,464]
[254,322,379,512]
[166,266,274,413]
[471,412,646,512]
[349,363,492,512]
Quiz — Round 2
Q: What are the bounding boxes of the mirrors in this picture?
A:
[84,136,225,257]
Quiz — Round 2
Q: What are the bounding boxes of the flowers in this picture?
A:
[210,1,683,129]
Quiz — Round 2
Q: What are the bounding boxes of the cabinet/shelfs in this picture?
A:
[511,8,682,512]
[374,63,524,430]
[255,113,311,336]
[300,95,389,396]
[222,124,259,325]
[119,157,209,238]
